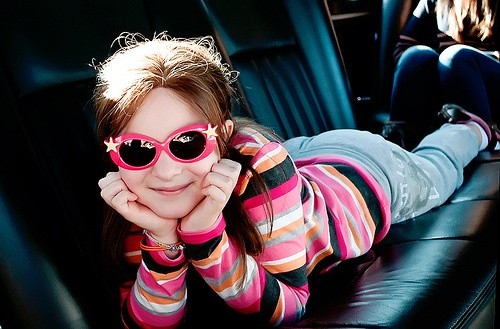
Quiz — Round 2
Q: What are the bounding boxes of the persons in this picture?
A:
[382,0,500,147]
[97,41,500,329]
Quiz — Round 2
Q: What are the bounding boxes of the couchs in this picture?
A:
[1,0,500,329]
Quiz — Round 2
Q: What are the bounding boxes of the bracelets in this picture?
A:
[139,238,182,251]
[140,228,184,251]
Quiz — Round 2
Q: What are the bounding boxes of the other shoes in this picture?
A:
[381,120,414,152]
[437,103,500,153]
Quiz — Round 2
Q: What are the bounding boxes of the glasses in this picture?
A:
[103,122,220,171]
